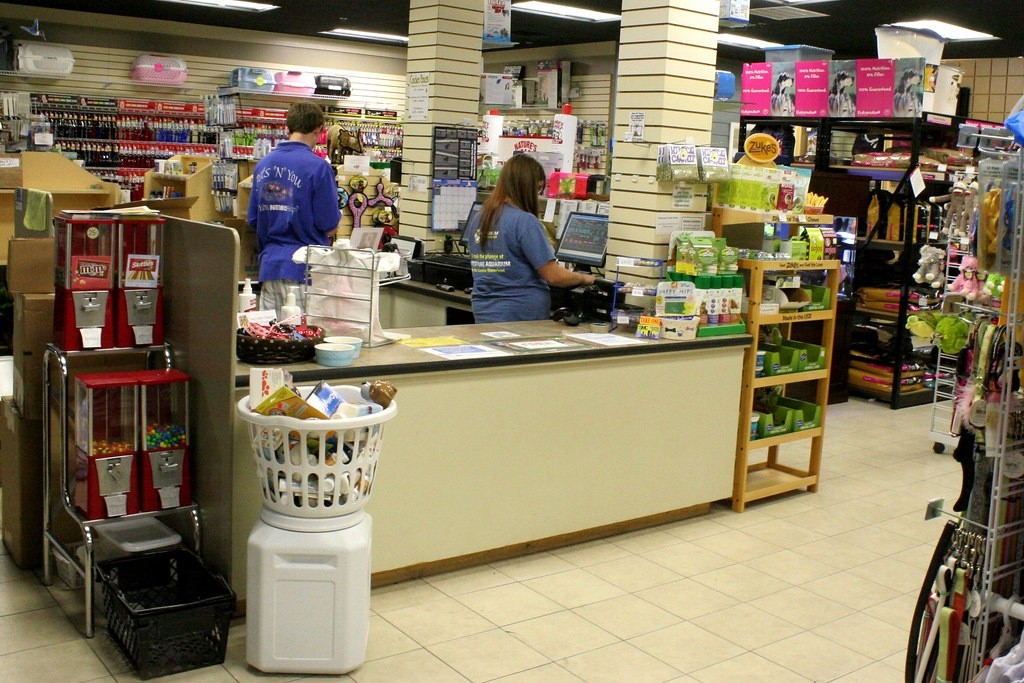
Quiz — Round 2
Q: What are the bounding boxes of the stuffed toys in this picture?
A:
[947,256,1005,300]
[913,244,946,288]
[906,312,968,354]
[929,181,978,237]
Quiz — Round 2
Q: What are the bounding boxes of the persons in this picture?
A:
[468,153,596,324]
[246,101,340,310]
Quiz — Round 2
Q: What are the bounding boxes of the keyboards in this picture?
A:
[427,254,472,269]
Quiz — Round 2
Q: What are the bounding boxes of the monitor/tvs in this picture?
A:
[458,201,485,247]
[554,211,609,276]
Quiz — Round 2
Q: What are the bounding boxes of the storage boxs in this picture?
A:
[483,0,511,43]
[719,0,750,28]
[229,67,353,97]
[875,27,965,116]
[722,222,838,439]
[249,368,292,411]
[132,55,188,84]
[761,44,836,63]
[0,236,148,570]
[480,60,571,105]
[14,43,75,72]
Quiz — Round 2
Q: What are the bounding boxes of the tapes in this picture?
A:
[590,323,609,334]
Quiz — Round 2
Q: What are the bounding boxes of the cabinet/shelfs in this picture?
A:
[609,257,663,332]
[31,101,289,172]
[712,155,841,513]
[738,113,1015,410]
[144,155,260,223]
[0,152,120,265]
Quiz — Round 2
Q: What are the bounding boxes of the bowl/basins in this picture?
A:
[323,336,363,359]
[314,343,355,366]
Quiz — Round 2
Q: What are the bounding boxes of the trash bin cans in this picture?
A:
[237,385,398,518]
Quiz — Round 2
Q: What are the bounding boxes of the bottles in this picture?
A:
[39,111,220,203]
[238,278,256,313]
